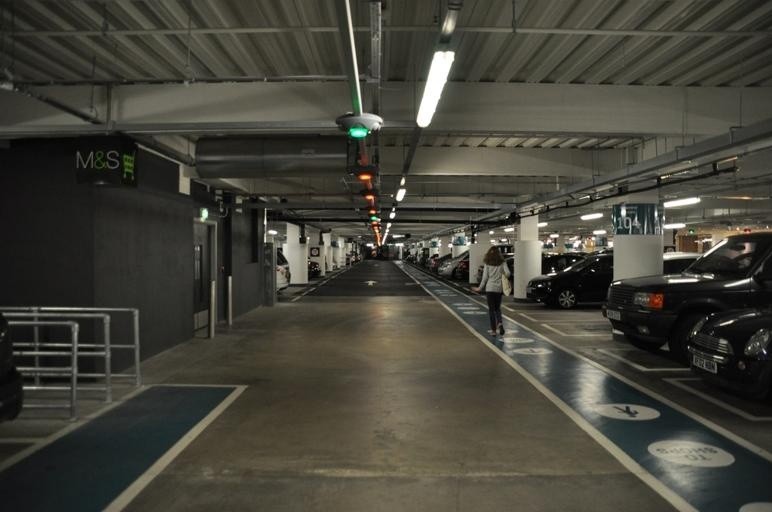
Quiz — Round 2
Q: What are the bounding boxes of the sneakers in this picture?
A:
[498,323,505,335]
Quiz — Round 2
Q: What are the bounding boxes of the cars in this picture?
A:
[264,243,360,294]
[1,313,25,424]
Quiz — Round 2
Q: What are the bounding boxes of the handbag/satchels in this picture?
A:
[501,271,512,297]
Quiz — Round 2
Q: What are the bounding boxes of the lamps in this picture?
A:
[332,108,384,140]
[415,42,456,131]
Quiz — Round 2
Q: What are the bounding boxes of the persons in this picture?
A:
[472,244,513,336]
